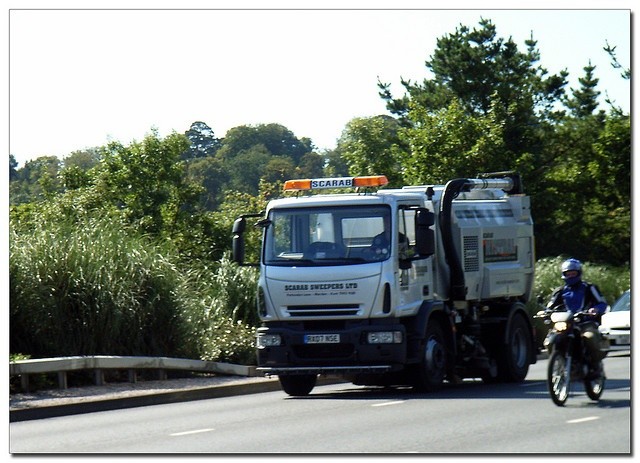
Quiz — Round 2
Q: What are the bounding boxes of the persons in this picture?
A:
[370,213,413,259]
[537,259,608,379]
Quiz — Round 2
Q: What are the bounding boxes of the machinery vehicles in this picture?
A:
[228,170,540,396]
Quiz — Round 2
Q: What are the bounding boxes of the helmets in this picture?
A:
[561,259,583,286]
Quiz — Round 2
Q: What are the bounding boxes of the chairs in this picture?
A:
[361,230,407,261]
[305,242,347,260]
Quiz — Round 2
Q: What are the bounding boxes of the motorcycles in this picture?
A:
[532,308,613,408]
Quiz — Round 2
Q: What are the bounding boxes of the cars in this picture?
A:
[597,287,630,354]
